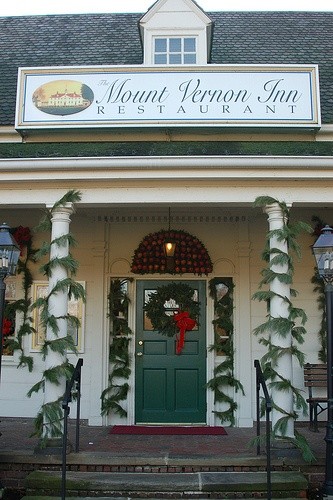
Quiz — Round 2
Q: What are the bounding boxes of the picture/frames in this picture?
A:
[27,279,88,355]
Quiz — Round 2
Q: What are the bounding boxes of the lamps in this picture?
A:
[161,207,177,259]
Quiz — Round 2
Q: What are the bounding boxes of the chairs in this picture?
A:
[302,362,328,434]
